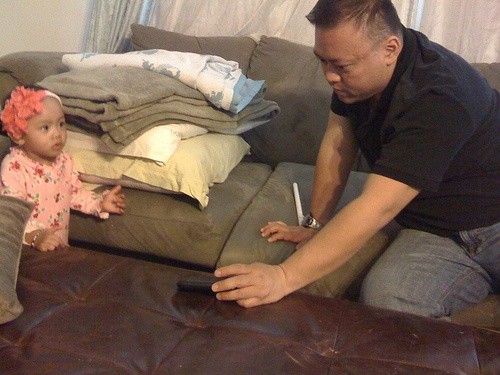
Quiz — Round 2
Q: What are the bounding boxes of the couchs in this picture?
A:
[0,23,500,332]
[0,245,500,375]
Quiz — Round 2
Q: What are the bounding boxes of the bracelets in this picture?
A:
[30,230,43,248]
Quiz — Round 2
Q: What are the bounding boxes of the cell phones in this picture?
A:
[176,275,224,291]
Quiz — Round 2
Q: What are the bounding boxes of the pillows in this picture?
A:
[0,195,36,326]
[62,129,252,211]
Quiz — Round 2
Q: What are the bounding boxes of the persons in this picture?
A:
[0,87,126,252]
[211,0,500,324]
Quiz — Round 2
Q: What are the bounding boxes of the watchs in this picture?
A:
[301,213,323,231]
